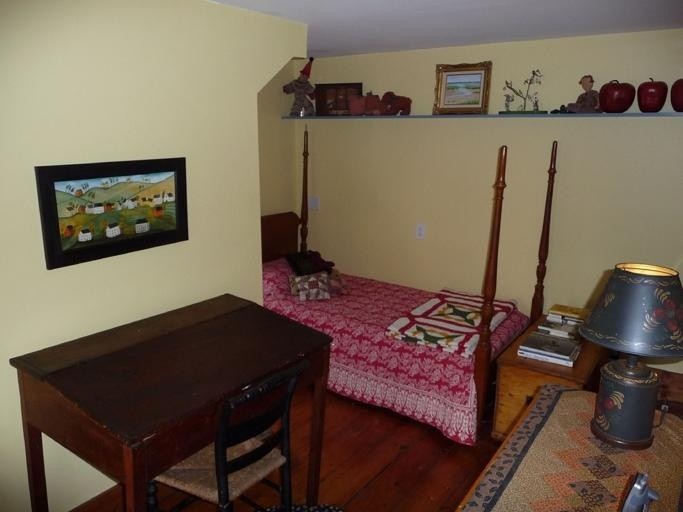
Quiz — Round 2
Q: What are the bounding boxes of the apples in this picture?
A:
[599,80,635,113]
[671,79,683,112]
[637,78,668,112]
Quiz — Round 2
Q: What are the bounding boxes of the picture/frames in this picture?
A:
[428,59,496,114]
[32,156,191,273]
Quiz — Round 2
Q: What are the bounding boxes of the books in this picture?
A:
[515,301,590,369]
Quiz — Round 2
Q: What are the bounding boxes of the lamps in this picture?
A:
[574,259,683,450]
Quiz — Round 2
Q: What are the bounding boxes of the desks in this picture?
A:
[452,382,683,511]
[8,292,334,512]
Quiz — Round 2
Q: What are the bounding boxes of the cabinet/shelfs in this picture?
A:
[635,361,683,411]
[490,301,600,442]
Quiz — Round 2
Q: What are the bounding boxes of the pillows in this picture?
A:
[286,269,351,297]
[294,268,331,300]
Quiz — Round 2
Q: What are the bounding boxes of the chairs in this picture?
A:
[144,355,316,511]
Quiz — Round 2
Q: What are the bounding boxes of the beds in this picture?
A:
[261,130,560,445]
